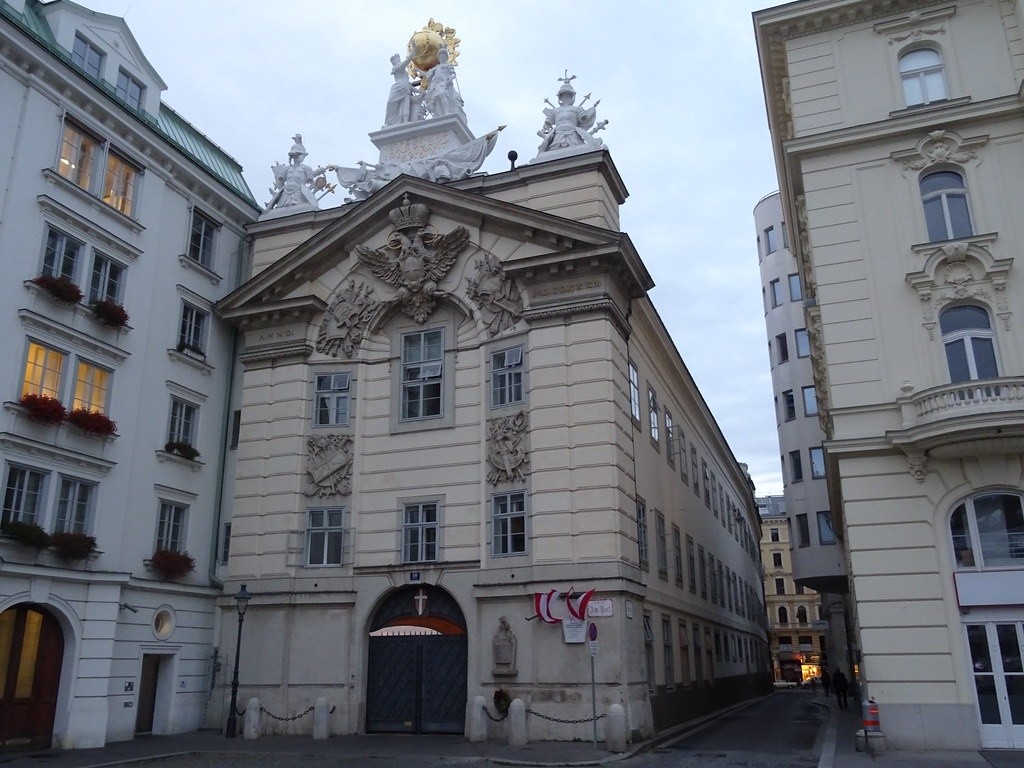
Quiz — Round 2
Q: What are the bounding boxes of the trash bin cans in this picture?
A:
[862,699,881,732]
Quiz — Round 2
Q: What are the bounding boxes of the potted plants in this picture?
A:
[165,442,201,460]
[177,340,207,363]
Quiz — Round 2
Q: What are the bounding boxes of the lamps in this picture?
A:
[731,501,736,512]
[119,602,139,614]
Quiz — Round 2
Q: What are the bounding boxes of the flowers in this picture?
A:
[36,270,86,306]
[69,408,118,436]
[3,521,49,544]
[52,533,97,560]
[19,393,68,426]
[153,550,197,584]
[92,300,130,331]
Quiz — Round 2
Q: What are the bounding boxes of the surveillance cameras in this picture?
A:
[124,603,139,613]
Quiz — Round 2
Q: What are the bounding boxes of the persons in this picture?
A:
[384,39,417,124]
[832,667,849,710]
[811,678,816,691]
[819,670,831,697]
[427,48,455,116]
